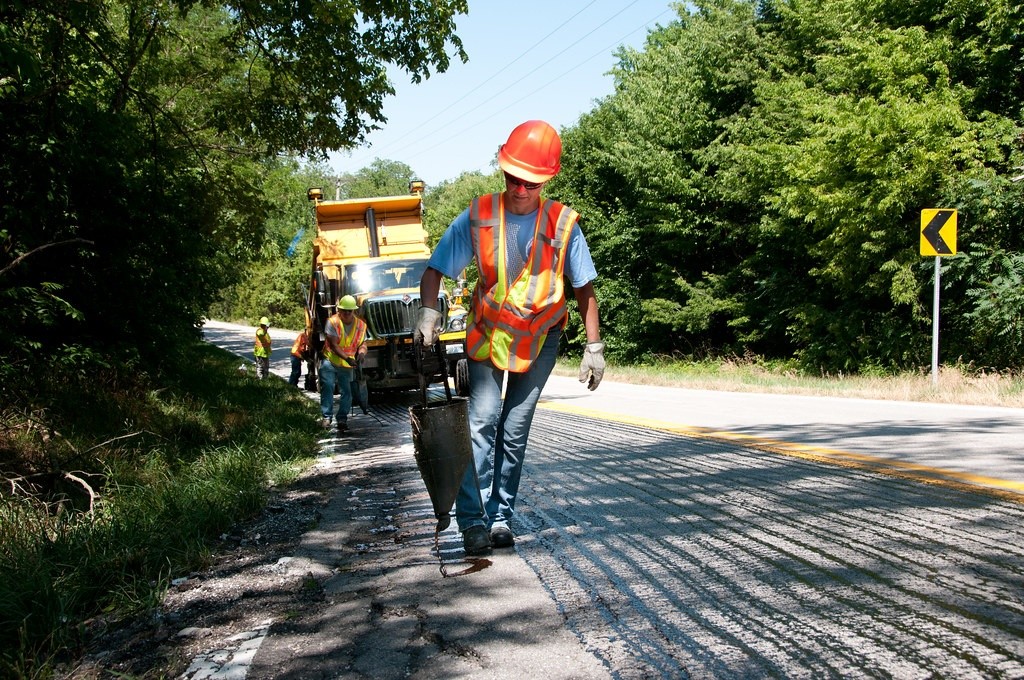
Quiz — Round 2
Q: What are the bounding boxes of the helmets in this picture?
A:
[498,120,561,183]
[337,295,359,310]
[259,317,270,325]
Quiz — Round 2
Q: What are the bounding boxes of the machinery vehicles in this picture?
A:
[304,181,472,397]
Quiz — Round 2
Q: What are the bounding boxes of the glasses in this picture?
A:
[504,172,543,189]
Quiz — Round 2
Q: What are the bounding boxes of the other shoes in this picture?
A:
[465,525,514,554]
[322,419,330,427]
[338,421,347,429]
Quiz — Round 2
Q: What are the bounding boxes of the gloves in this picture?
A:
[579,342,605,391]
[356,353,365,364]
[347,357,358,366]
[414,306,442,346]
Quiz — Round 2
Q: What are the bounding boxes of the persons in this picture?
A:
[254,316,271,380]
[317,294,367,431]
[412,121,606,552]
[289,332,309,387]
